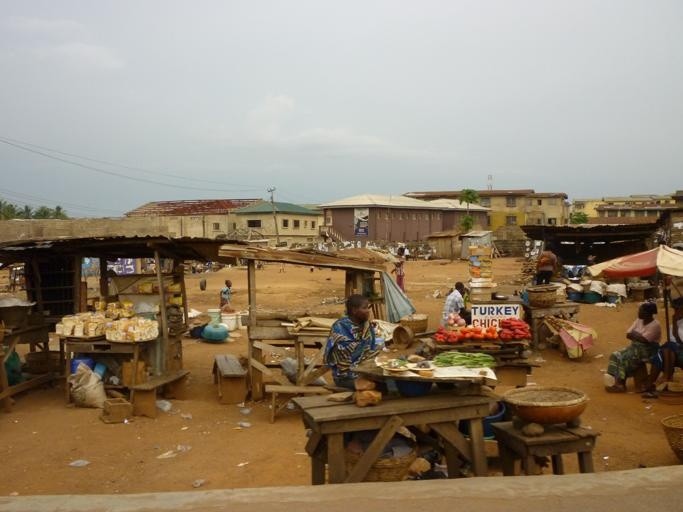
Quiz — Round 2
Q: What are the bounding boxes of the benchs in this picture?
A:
[213,354,247,405]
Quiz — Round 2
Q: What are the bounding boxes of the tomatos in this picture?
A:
[434,324,499,344]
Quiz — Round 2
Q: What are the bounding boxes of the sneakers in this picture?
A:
[605,385,626,393]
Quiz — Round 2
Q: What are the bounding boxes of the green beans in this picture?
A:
[432,352,498,369]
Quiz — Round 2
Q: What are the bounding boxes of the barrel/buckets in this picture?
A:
[71,357,93,374]
[221,312,240,331]
[207,308,221,323]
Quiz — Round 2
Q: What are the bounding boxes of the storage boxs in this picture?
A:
[122,361,145,386]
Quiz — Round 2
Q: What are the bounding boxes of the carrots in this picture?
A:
[500,317,532,341]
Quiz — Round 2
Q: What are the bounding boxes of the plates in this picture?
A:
[380,360,435,372]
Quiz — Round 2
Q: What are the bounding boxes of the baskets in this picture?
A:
[660,414,683,462]
[400,313,428,333]
[526,285,557,308]
[501,384,587,424]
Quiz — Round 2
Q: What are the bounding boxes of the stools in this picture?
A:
[490,420,600,474]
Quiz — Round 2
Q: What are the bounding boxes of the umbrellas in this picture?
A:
[584,244,683,343]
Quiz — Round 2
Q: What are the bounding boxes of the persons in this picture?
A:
[648,296,683,387]
[534,246,557,286]
[440,279,471,327]
[604,302,662,392]
[390,247,405,293]
[219,279,233,311]
[321,294,388,398]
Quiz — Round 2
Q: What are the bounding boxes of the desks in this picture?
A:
[62,341,149,406]
[292,391,503,481]
[519,298,579,351]
